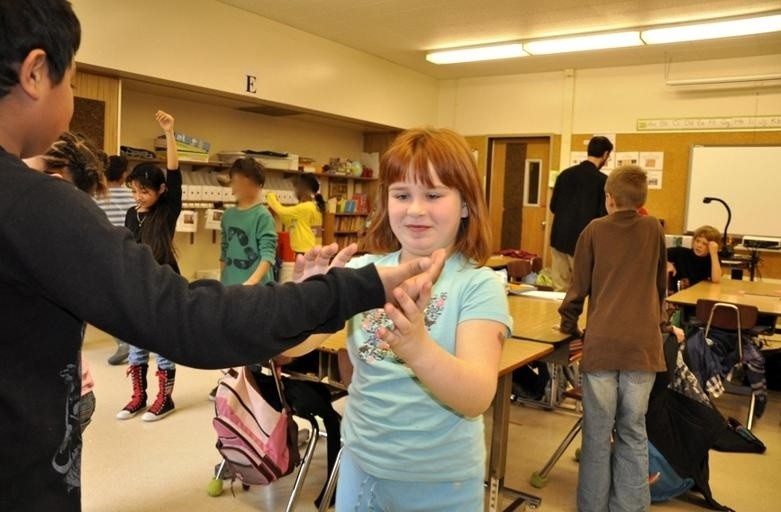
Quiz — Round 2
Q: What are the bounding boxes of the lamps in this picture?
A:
[425,11,781,65]
[703,197,732,257]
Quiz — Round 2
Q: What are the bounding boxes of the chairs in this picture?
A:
[531,386,582,488]
[318,348,353,512]
[531,258,542,272]
[696,299,757,430]
[207,354,347,512]
[508,261,532,282]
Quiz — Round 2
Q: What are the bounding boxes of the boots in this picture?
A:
[140,367,175,422]
[107,339,130,365]
[115,364,147,420]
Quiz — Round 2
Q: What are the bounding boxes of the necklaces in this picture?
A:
[137,209,146,227]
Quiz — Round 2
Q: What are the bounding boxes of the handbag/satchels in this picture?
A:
[611,425,694,504]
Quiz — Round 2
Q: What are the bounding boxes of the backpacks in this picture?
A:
[212,365,300,498]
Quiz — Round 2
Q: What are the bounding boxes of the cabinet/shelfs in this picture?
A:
[120,154,324,209]
[325,173,381,253]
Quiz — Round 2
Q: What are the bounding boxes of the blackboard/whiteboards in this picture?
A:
[682,143,781,238]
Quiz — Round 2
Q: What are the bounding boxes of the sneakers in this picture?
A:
[208,368,237,401]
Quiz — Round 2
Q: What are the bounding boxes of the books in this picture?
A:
[328,193,374,255]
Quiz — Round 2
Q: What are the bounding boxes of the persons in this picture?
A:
[91,155,138,366]
[552,137,614,292]
[552,163,685,512]
[22,131,111,203]
[292,127,513,512]
[266,173,325,260]
[664,225,723,292]
[116,110,182,421]
[0,0,446,512]
[209,157,279,401]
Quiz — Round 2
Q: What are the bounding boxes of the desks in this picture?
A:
[664,274,781,326]
[277,321,555,512]
[476,254,528,269]
[508,295,587,414]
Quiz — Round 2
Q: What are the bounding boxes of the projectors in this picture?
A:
[741,235,781,249]
